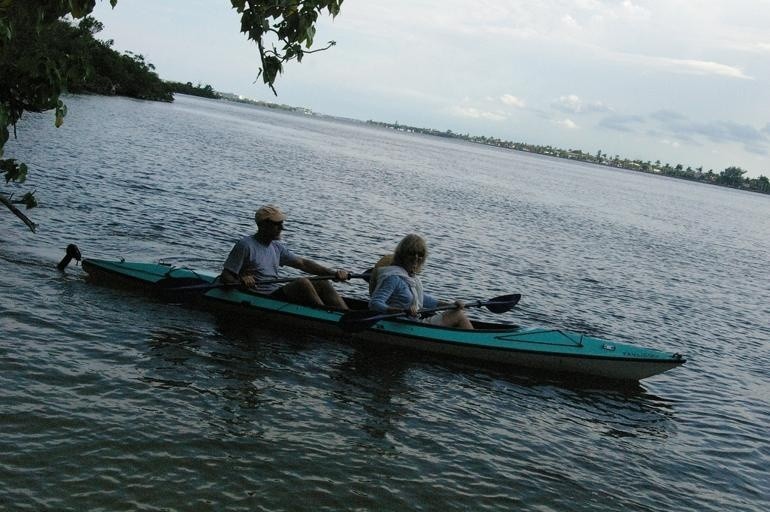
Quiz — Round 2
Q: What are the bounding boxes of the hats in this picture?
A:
[255,204,286,224]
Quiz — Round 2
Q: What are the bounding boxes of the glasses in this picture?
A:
[407,249,425,257]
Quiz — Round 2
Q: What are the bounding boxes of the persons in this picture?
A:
[368,234,475,330]
[221,206,349,310]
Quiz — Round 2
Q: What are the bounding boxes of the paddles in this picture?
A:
[339,295,521,333]
[155,267,374,307]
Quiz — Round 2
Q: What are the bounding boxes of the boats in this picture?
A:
[81,257,687,381]
